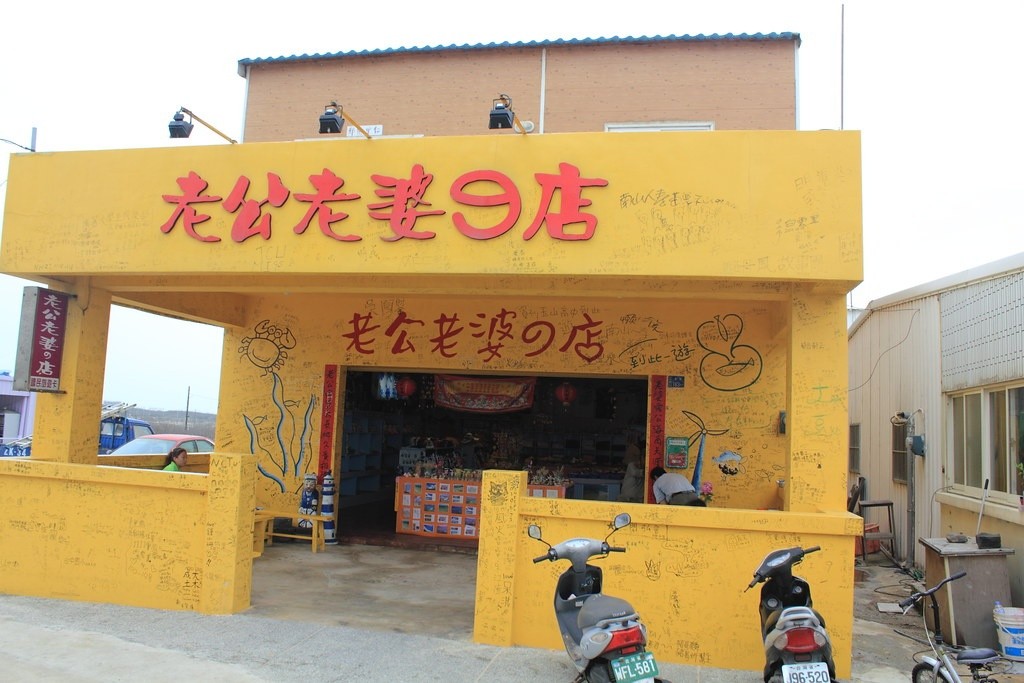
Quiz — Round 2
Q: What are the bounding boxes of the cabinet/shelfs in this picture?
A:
[919,537,1015,649]
[336,395,645,529]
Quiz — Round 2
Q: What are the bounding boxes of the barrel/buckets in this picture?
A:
[993,607,1024,661]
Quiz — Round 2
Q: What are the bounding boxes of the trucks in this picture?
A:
[0,417,156,457]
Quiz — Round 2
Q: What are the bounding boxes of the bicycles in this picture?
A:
[898,572,999,683]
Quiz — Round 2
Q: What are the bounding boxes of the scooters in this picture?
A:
[743,545,840,683]
[527,513,672,683]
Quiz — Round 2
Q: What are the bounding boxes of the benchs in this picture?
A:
[256,510,335,553]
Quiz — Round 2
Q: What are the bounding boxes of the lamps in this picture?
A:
[319,102,371,140]
[488,93,527,134]
[168,107,238,145]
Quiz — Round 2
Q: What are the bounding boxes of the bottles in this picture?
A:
[994,601,1005,614]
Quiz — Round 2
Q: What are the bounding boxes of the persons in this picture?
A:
[650,466,696,505]
[162,447,188,472]
[624,440,646,471]
[619,461,645,502]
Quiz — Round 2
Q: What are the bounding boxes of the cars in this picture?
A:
[106,434,214,455]
